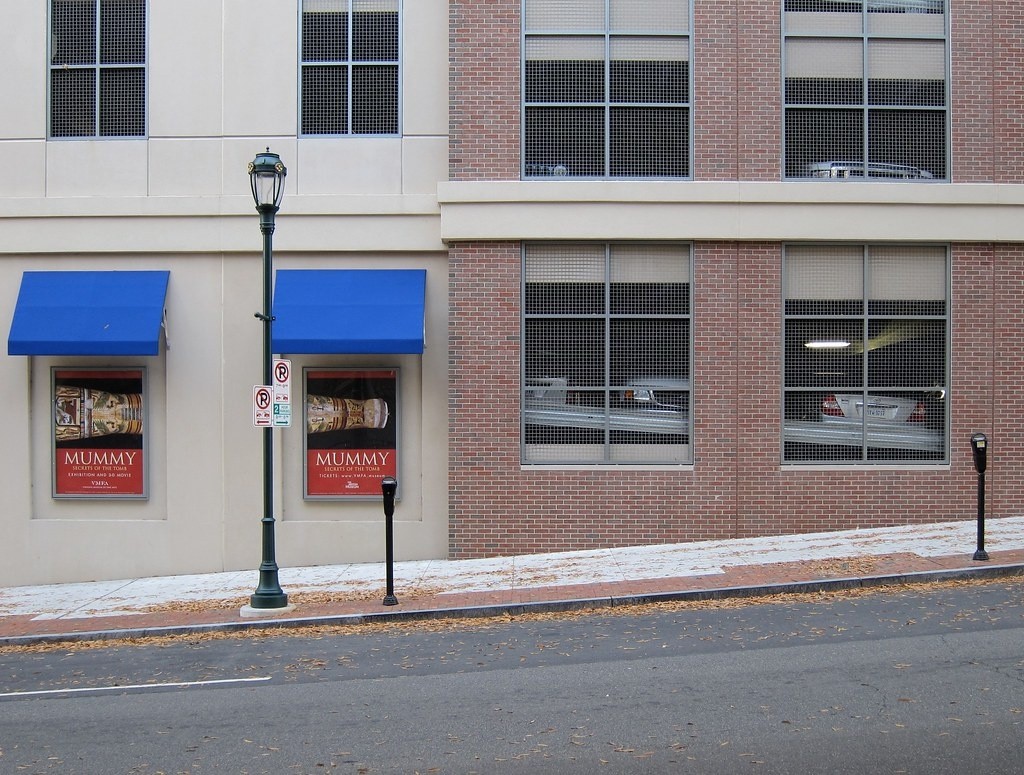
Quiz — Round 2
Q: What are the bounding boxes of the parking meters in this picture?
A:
[381,475,399,606]
[969,432,989,560]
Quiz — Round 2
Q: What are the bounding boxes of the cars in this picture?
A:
[619,362,689,413]
[819,379,927,430]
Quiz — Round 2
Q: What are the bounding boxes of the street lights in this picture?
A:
[250,146,289,609]
